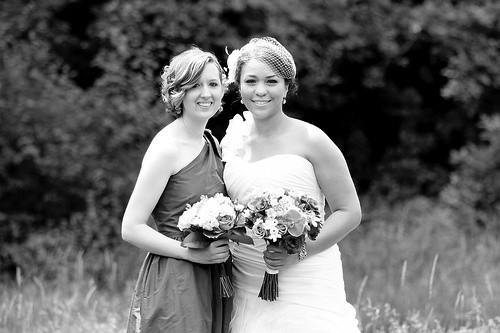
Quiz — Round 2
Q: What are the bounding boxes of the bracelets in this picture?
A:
[297,243,308,261]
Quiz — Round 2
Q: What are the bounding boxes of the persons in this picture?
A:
[222,36,365,332]
[121,45,241,333]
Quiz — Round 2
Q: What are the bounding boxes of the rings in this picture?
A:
[223,258,226,263]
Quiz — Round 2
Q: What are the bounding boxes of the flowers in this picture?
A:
[177,193,255,298]
[245,188,324,301]
